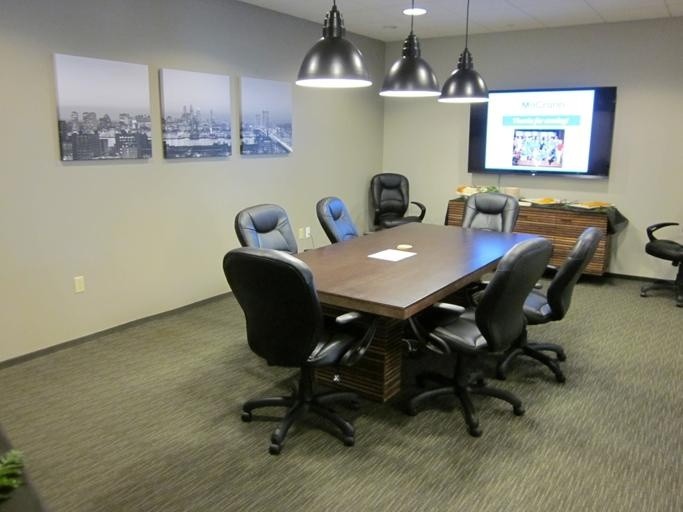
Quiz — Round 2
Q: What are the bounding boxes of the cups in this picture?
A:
[503,187,520,204]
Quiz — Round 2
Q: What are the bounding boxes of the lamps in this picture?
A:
[378,0,441,99]
[296,1,372,89]
[437,0,489,103]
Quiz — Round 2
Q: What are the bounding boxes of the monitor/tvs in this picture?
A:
[468,84,617,178]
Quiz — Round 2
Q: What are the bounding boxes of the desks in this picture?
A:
[292,221,538,404]
[445,198,628,281]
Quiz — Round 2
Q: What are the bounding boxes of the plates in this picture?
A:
[520,196,571,209]
[565,200,615,210]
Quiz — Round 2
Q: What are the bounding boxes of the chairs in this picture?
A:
[493,226,603,385]
[223,248,375,455]
[369,172,426,230]
[314,196,361,244]
[639,222,682,306]
[235,203,300,255]
[459,193,519,235]
[400,238,553,439]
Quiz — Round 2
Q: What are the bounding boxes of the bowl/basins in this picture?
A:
[454,184,490,200]
